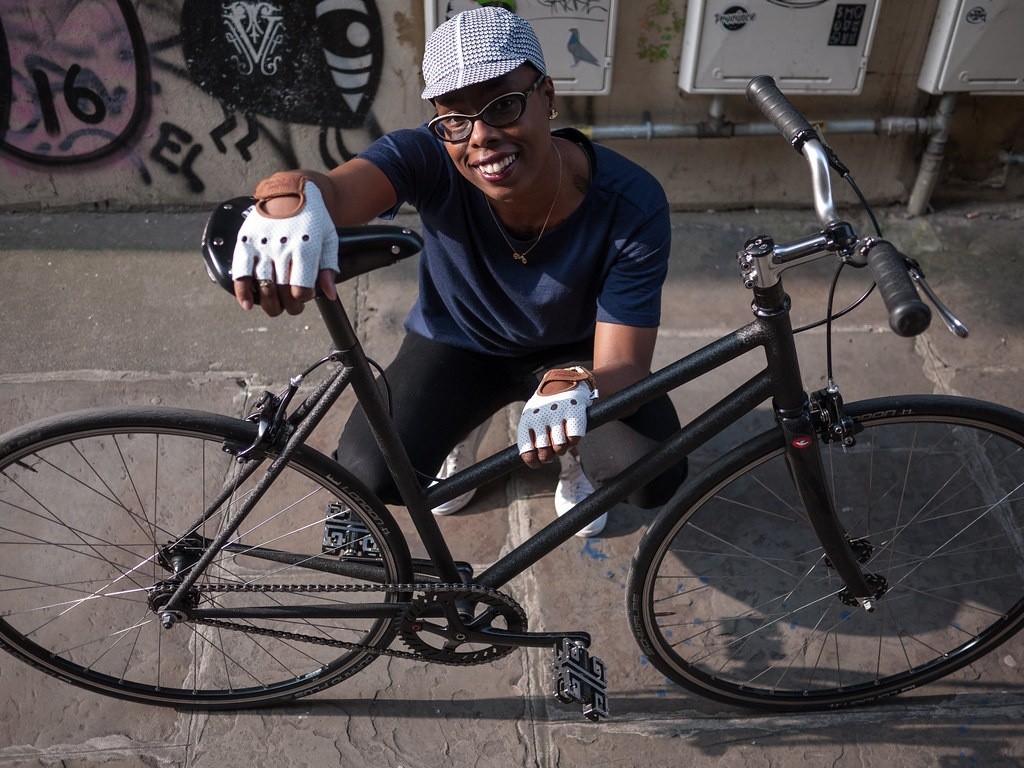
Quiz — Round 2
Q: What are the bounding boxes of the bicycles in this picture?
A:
[1,72,1024,726]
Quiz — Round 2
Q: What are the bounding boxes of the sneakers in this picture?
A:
[426,417,493,516]
[554,447,608,538]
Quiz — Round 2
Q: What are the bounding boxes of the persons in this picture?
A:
[232,6,688,537]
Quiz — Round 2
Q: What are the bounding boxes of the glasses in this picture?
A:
[427,72,545,144]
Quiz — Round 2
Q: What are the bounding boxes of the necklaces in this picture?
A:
[575,140,594,190]
[484,140,562,265]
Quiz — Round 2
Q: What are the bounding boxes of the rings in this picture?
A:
[258,280,273,286]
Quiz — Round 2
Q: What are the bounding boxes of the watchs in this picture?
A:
[563,365,598,387]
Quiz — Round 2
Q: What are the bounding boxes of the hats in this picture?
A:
[419,6,546,101]
